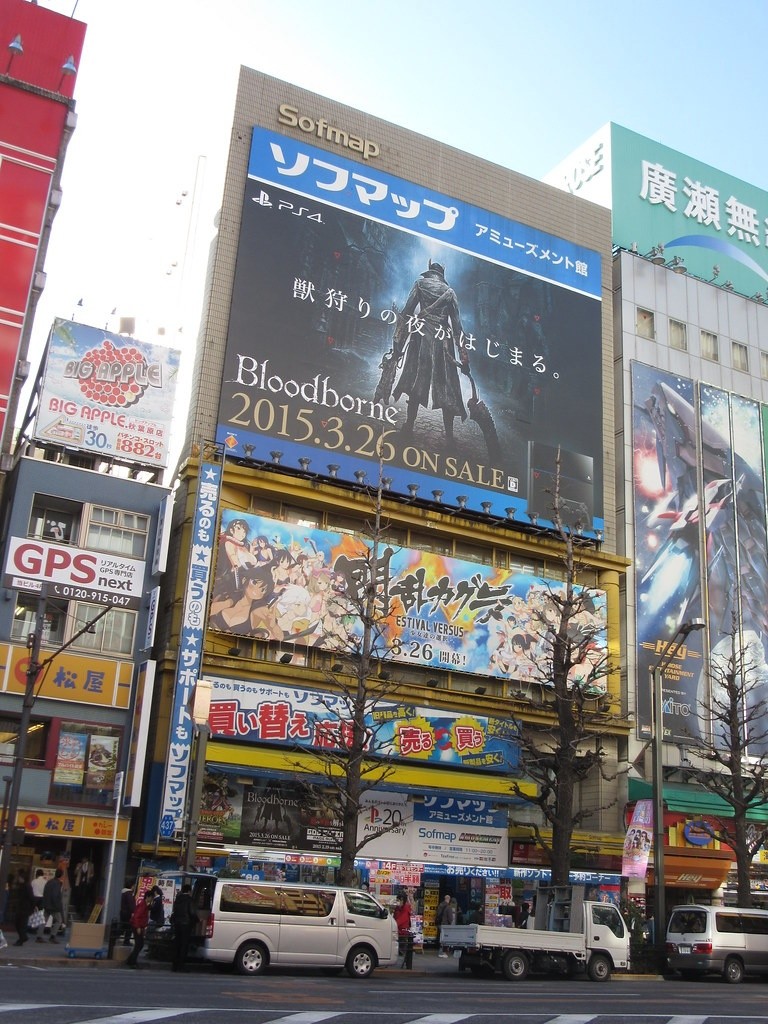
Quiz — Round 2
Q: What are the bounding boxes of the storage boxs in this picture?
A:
[68,923,104,949]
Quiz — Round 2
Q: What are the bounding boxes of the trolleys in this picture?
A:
[64,944,109,960]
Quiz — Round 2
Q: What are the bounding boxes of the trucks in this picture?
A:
[441,900,637,982]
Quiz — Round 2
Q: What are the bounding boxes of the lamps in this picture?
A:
[407,483,420,496]
[528,512,603,540]
[227,648,242,656]
[599,704,611,713]
[456,495,469,509]
[332,665,343,672]
[379,671,390,678]
[354,470,367,483]
[280,653,293,664]
[270,451,284,464]
[431,490,444,503]
[427,680,487,694]
[242,443,255,458]
[505,507,517,519]
[298,457,312,471]
[16,604,26,616]
[382,477,392,490]
[480,502,493,514]
[327,464,340,478]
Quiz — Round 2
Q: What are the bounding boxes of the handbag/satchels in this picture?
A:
[27,907,46,928]
[435,913,443,925]
[45,915,53,928]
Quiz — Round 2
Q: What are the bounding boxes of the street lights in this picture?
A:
[655,614,709,977]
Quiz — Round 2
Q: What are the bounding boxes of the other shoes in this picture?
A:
[0,940,8,949]
[13,939,23,946]
[22,934,29,943]
[438,954,448,959]
[128,961,138,969]
[172,966,179,972]
[36,937,47,943]
[49,938,60,945]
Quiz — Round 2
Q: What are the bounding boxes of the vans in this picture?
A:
[664,903,768,983]
[158,869,400,980]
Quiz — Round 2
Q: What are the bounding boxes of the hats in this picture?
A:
[449,897,457,906]
[522,903,529,910]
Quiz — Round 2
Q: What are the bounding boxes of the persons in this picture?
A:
[625,829,650,860]
[393,263,470,452]
[518,902,530,929]
[434,895,483,959]
[393,893,411,954]
[74,855,94,917]
[121,881,194,973]
[0,868,66,949]
